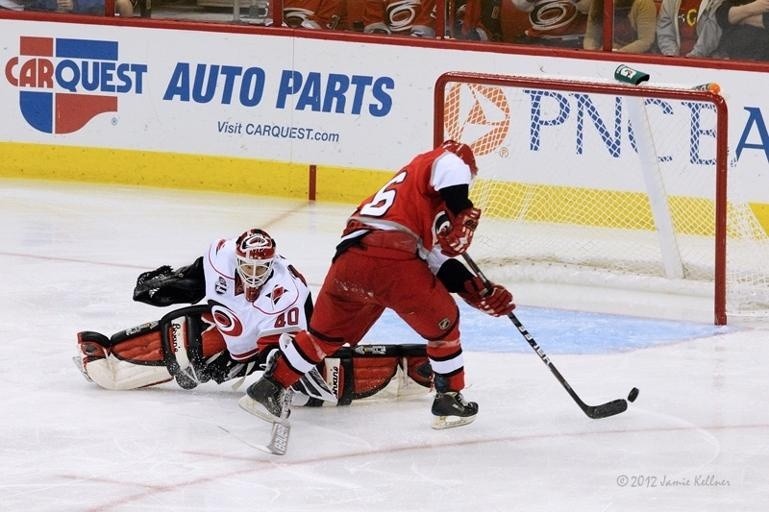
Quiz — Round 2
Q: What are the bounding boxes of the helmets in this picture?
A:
[441,140,477,177]
[236,229,276,287]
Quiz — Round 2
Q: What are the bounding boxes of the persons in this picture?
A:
[76,228,326,409]
[246,139,517,418]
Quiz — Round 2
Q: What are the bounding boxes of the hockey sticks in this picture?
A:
[461,250,627,418]
[218,389,292,455]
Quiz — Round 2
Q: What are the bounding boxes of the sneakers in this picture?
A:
[432,392,478,417]
[247,376,290,418]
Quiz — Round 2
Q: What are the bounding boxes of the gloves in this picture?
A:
[473,285,515,317]
[438,209,481,256]
